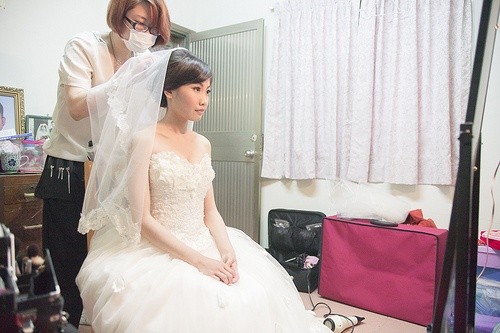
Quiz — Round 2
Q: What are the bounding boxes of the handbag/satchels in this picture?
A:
[34,167,85,201]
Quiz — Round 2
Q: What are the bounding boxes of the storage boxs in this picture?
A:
[26,115,52,140]
[20,140,43,173]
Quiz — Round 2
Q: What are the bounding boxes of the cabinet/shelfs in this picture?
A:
[0,174,43,257]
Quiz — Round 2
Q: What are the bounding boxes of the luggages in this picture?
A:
[264,208,326,293]
[318,214,448,326]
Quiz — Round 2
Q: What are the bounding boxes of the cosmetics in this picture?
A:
[18,245,46,289]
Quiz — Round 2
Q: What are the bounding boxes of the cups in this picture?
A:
[1,150,28,173]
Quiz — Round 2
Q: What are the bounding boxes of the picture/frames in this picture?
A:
[0,85,26,137]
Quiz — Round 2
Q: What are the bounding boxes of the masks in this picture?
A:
[117,21,157,52]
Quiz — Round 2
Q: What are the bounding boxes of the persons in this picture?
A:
[37,120,55,140]
[76,47,336,333]
[35,0,171,328]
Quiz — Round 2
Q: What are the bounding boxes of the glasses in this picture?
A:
[124,15,161,36]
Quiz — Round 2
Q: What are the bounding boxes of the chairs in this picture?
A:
[84,159,95,252]
